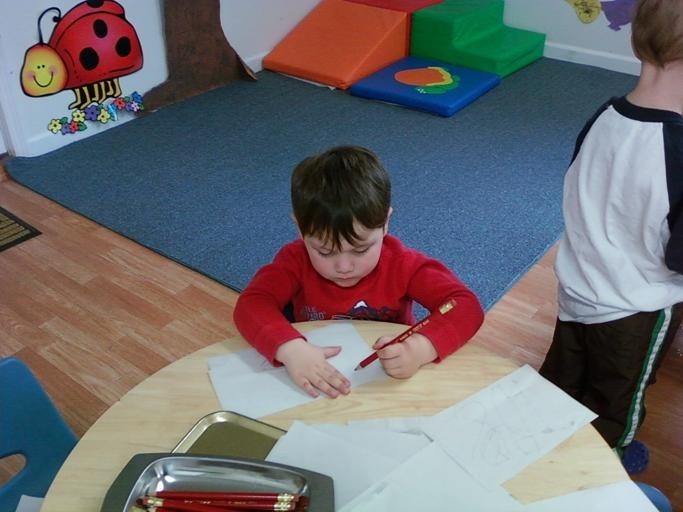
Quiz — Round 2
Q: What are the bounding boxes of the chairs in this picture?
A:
[0,356,78,512]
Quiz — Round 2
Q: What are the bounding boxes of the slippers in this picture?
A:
[622,440,648,474]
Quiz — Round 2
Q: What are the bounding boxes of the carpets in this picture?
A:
[3,55,642,312]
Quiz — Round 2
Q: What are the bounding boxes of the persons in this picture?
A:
[533,0,683,471]
[234,146,484,399]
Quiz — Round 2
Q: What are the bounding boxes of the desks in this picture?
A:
[39,319,660,512]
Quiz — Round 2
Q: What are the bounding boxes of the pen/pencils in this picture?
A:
[134,491,296,510]
[354,299,456,372]
